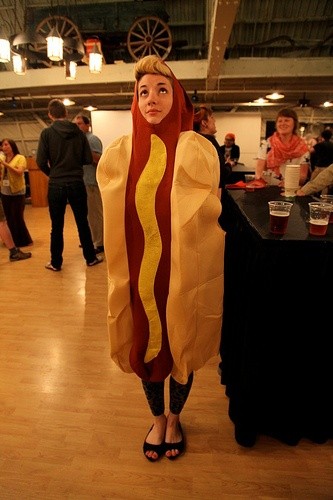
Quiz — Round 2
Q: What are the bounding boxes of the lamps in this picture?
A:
[266,84,284,100]
[320,102,333,107]
[254,96,269,103]
[0,0,103,111]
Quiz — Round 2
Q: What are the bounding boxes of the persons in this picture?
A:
[280,164,333,196]
[95,55,226,463]
[36,99,104,271]
[71,109,103,255]
[190,106,235,189]
[219,133,241,167]
[256,109,311,188]
[309,130,333,168]
[0,199,32,262]
[0,137,34,248]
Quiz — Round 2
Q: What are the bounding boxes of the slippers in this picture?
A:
[165,421,185,460]
[143,425,164,462]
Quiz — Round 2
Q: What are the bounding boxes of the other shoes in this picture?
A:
[48,262,58,271]
[94,246,104,254]
[88,256,103,266]
[10,249,31,261]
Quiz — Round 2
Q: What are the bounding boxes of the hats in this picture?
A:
[225,134,235,140]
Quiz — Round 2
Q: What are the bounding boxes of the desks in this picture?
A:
[231,165,256,183]
[217,187,333,450]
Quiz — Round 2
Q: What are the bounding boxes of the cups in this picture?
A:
[309,203,332,238]
[283,165,300,201]
[320,194,333,223]
[262,173,271,185]
[268,201,294,236]
[245,175,255,192]
